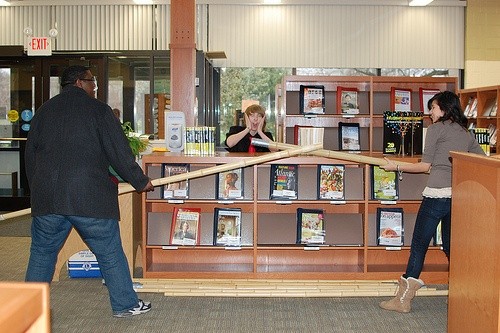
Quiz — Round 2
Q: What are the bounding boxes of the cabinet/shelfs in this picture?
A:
[0,282,51,333]
[52,75,500,333]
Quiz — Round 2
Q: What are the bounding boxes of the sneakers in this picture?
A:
[113,299,151,317]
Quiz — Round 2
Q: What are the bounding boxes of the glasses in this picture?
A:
[79,74,97,83]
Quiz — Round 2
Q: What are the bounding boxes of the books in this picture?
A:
[301,212,324,245]
[394,90,411,112]
[379,211,402,246]
[219,168,241,199]
[270,164,298,200]
[185,127,217,155]
[423,90,440,115]
[463,95,498,153]
[216,210,242,246]
[319,165,345,201]
[374,166,397,200]
[296,85,361,150]
[172,211,199,246]
[164,165,188,200]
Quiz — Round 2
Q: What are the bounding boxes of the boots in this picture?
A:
[378,273,425,313]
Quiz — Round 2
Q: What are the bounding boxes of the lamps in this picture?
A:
[24,22,59,56]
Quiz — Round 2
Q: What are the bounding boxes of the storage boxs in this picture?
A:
[67,251,103,278]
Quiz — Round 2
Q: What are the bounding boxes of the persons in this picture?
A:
[225,104,278,153]
[24,65,154,319]
[379,91,485,313]
